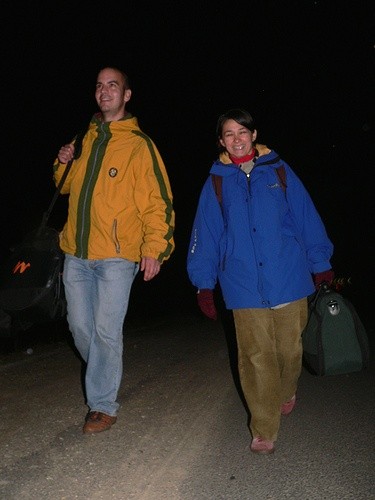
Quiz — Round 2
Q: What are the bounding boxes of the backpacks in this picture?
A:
[302,281,370,378]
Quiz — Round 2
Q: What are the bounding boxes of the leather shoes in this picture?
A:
[82,410,121,433]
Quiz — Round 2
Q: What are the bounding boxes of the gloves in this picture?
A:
[314,269,336,287]
[197,289,218,320]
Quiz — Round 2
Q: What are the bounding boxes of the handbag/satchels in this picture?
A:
[0,228,67,325]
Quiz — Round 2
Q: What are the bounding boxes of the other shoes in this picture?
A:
[250,436,276,454]
[282,394,298,414]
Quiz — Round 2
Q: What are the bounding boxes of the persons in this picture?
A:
[52,68,176,436]
[186,109,342,455]
